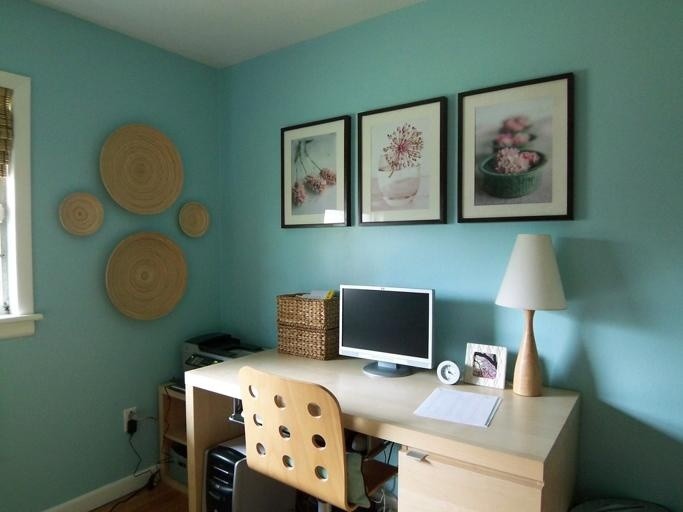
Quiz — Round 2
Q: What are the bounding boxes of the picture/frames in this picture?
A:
[355,95,448,227]
[279,114,351,229]
[461,342,507,389]
[456,71,576,223]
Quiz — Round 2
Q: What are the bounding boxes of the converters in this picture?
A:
[127,420,138,434]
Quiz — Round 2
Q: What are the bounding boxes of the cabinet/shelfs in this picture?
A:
[159,379,188,497]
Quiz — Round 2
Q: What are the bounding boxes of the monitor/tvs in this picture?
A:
[338,284,435,379]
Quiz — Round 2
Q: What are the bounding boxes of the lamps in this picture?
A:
[494,233,567,395]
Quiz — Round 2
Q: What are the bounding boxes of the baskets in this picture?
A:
[276,323,343,362]
[273,291,340,332]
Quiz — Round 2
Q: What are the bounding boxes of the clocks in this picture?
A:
[437,361,461,385]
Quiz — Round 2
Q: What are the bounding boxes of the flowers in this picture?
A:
[493,146,537,175]
[380,120,423,169]
[292,139,337,207]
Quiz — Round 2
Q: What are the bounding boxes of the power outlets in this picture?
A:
[123,406,137,432]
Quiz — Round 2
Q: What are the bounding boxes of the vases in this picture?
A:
[376,167,418,199]
[481,150,548,199]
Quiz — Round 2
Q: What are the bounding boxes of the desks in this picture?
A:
[183,345,583,512]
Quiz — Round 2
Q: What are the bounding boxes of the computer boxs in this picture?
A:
[202,436,297,512]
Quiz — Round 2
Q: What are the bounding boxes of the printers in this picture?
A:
[182,333,266,374]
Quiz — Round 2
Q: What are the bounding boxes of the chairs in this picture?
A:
[239,365,398,512]
